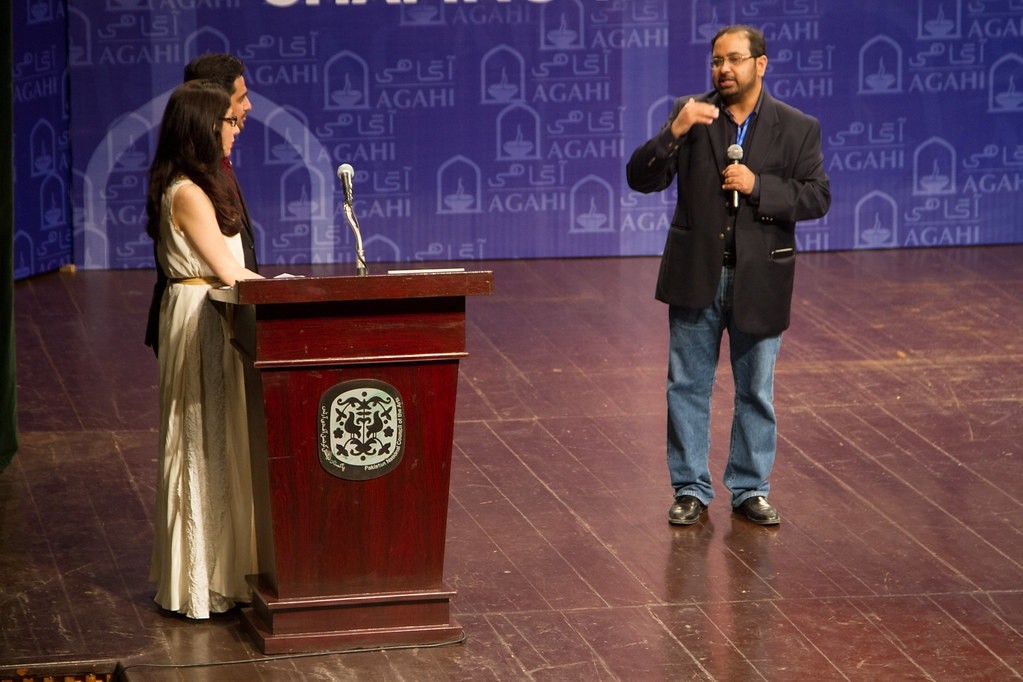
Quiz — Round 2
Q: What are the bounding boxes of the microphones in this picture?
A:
[337,163,354,205]
[727,144,744,209]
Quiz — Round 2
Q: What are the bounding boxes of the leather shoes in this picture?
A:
[668,496,709,524]
[733,496,781,525]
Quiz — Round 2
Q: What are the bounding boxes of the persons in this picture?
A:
[625,26,831,526]
[144,52,262,357]
[144,80,273,620]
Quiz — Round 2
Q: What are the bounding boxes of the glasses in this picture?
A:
[710,55,754,68]
[217,115,238,127]
